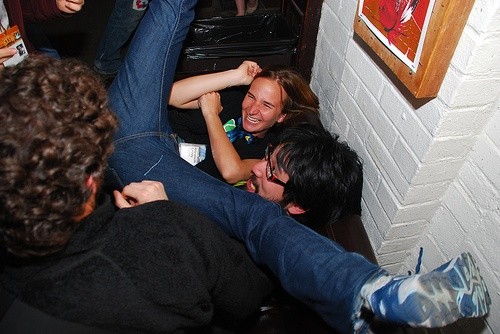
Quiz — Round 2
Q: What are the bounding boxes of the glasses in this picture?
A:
[265,145,286,187]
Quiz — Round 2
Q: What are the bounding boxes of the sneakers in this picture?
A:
[371,247,490,328]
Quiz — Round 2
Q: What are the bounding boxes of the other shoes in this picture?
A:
[247,0,257,14]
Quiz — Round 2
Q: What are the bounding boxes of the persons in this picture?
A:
[17,46,26,56]
[0,0,493,334]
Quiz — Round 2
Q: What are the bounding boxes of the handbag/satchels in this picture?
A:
[184,13,298,61]
